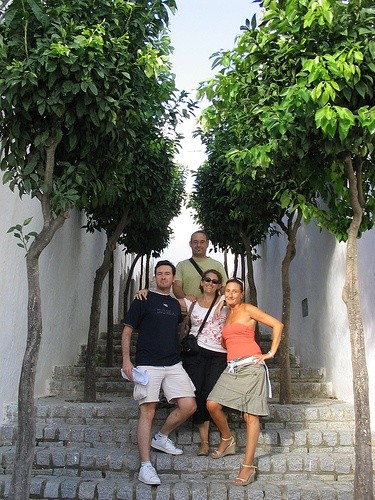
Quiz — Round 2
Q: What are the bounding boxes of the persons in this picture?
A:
[134,269,232,455]
[205,277,285,486]
[121,260,198,484]
[172,231,230,321]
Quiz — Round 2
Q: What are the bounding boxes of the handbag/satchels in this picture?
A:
[181,334,199,356]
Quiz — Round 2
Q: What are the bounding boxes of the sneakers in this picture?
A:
[150,434,183,455]
[138,464,161,485]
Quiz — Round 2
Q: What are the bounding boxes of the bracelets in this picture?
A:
[269,351,274,359]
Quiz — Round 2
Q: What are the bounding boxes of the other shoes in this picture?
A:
[198,441,209,456]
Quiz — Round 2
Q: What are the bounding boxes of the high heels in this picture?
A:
[212,436,236,458]
[233,465,257,485]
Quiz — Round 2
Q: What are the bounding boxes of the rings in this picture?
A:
[136,293,138,294]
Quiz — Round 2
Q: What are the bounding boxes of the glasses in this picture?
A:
[229,277,244,292]
[202,277,220,285]
